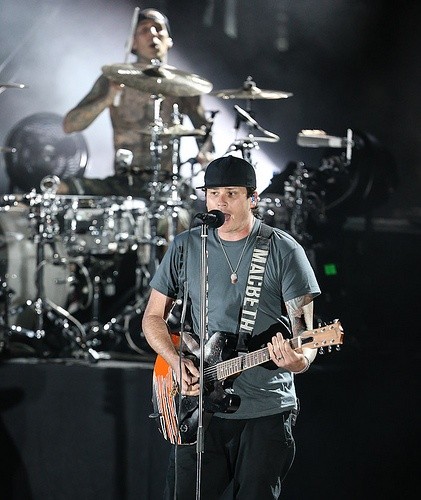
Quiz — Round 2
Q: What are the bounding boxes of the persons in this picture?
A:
[54,11,216,253]
[142,155,321,500]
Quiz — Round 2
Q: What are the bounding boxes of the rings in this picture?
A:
[277,356,283,360]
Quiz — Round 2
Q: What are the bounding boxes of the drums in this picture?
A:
[0,206,70,341]
[139,198,205,267]
[62,252,156,362]
[57,195,151,258]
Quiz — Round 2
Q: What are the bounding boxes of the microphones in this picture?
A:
[234,105,262,130]
[195,210,225,229]
[298,131,355,150]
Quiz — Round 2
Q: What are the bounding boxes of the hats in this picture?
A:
[196,154,256,189]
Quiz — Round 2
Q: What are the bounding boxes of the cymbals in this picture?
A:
[209,85,294,101]
[134,123,208,141]
[97,58,217,103]
[0,79,29,89]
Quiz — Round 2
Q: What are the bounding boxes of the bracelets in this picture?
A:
[292,356,310,374]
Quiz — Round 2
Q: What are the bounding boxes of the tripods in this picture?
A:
[0,98,198,362]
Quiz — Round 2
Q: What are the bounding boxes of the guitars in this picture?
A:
[152,316,345,446]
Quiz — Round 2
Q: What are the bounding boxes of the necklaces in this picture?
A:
[219,217,254,284]
